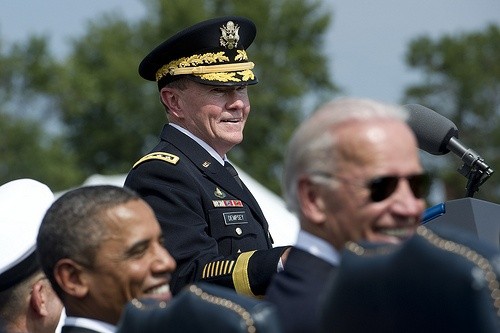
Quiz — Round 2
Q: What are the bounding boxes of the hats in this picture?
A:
[0,178,53,284]
[138,16,258,85]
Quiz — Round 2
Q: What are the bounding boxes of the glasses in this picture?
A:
[311,172,433,202]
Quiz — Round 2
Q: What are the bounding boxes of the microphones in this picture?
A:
[402,104,494,174]
